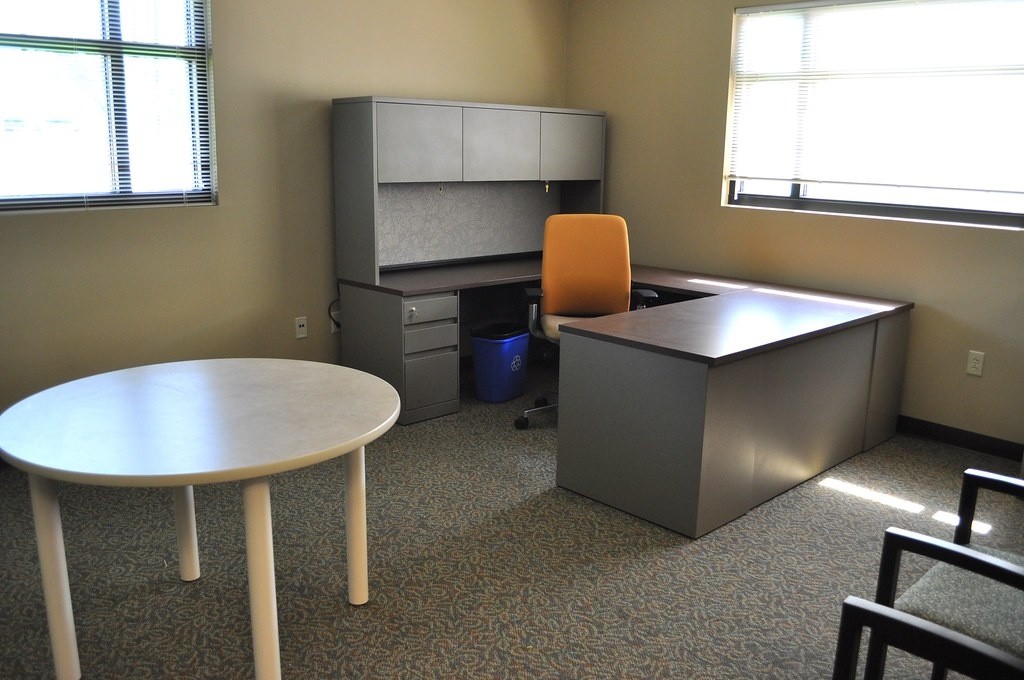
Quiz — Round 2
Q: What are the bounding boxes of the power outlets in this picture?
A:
[295,316,307,339]
[965,350,985,376]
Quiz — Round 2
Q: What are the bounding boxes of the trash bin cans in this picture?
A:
[468,322,531,403]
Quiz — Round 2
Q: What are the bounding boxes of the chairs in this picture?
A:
[833,467,1024,680]
[515,214,658,429]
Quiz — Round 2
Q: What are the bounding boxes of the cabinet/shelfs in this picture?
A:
[332,96,606,285]
[336,290,462,425]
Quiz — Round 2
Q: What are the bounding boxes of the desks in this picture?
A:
[381,252,914,540]
[0,358,401,680]
